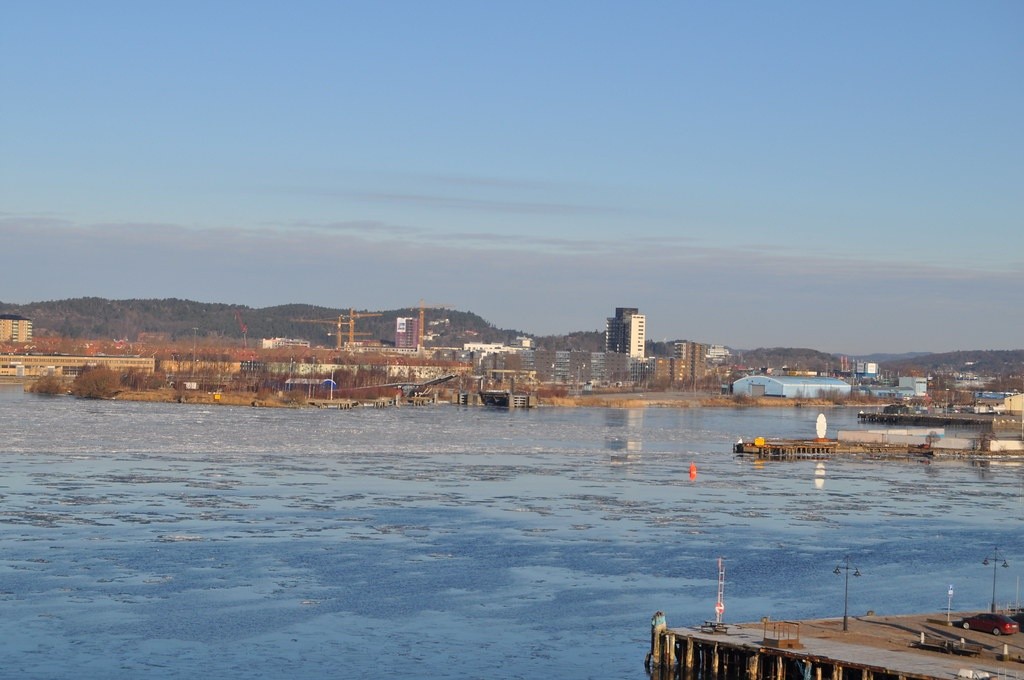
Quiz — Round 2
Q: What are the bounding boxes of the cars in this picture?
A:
[959,612,1020,636]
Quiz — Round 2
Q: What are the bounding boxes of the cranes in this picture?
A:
[402,297,451,347]
[287,307,385,352]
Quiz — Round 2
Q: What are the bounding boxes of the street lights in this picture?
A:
[833,554,862,631]
[982,547,1009,614]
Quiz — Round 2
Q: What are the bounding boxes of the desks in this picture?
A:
[701,621,729,635]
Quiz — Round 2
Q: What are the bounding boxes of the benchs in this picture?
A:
[712,627,728,629]
[701,625,716,627]
